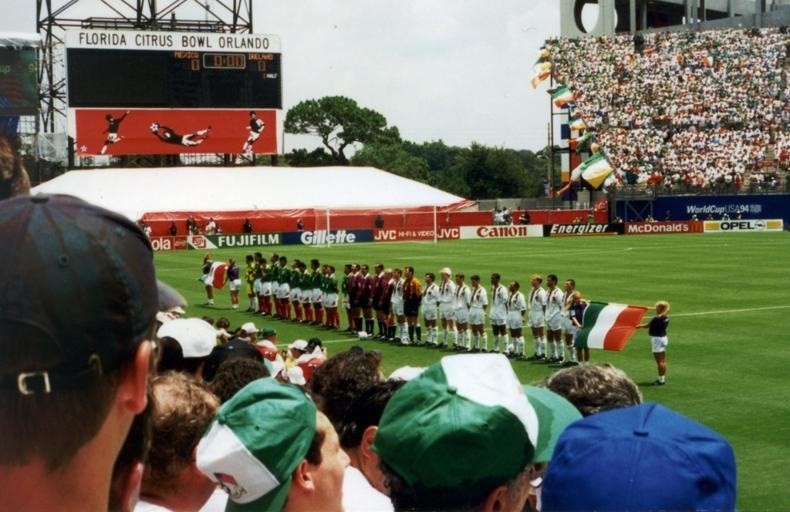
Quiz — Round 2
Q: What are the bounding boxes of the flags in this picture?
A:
[568,132,597,153]
[530,63,552,88]
[197,262,227,289]
[547,83,575,107]
[581,151,613,189]
[572,300,648,351]
[568,112,586,131]
[533,47,552,64]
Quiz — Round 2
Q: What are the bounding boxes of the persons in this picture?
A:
[98,110,132,156]
[545,26,790,198]
[240,111,267,152]
[2,128,745,512]
[150,121,214,146]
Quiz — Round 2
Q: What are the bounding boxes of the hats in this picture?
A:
[192,378,315,512]
[240,322,277,337]
[372,351,586,488]
[202,341,263,379]
[1,192,187,394]
[542,402,736,512]
[288,338,321,353]
[156,317,217,358]
[439,268,451,275]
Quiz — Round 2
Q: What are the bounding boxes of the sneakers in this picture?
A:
[652,380,665,385]
[246,308,291,321]
[205,302,213,305]
[231,307,239,311]
[529,353,579,366]
[291,318,340,330]
[343,327,396,341]
[398,337,448,349]
[451,343,525,360]
